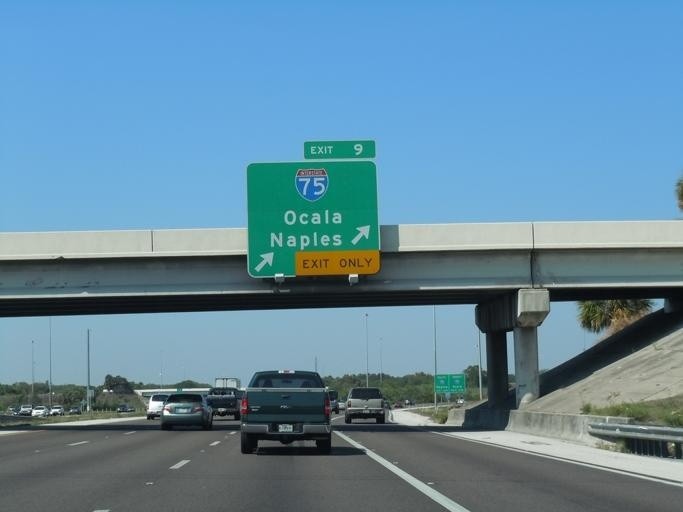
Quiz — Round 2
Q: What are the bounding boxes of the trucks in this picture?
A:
[214,377,241,389]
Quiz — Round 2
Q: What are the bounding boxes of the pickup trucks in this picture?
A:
[238,370,337,456]
[206,387,240,422]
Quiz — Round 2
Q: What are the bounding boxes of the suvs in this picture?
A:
[328,390,339,415]
[49,404,65,416]
[344,387,386,425]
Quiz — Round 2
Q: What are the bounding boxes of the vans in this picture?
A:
[145,393,172,420]
[455,395,464,403]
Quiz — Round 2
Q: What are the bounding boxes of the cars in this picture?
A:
[116,404,135,412]
[31,406,49,417]
[68,407,82,415]
[337,400,346,410]
[382,398,416,408]
[160,392,214,430]
[8,404,34,416]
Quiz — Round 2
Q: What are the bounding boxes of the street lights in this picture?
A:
[364,313,369,387]
[30,339,36,397]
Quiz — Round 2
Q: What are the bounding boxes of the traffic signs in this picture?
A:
[433,373,466,394]
